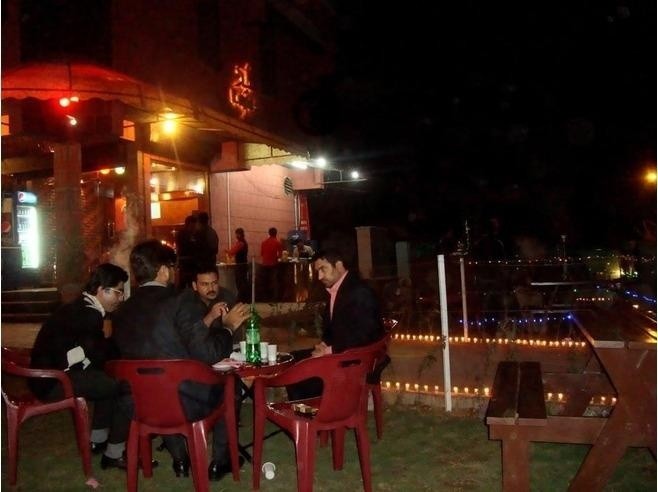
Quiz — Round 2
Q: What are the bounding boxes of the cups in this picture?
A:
[238,341,246,354]
[261,462,275,480]
[259,342,268,359]
[267,345,277,362]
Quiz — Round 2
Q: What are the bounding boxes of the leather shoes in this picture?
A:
[207,456,244,481]
[100,451,159,471]
[89,438,109,454]
[172,458,190,478]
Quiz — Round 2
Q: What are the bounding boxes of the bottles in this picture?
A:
[245,307,260,363]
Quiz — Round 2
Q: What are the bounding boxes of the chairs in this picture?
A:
[319,335,392,448]
[252,339,387,491]
[102,356,240,492]
[1,344,94,485]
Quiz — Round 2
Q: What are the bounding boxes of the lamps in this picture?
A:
[312,153,330,171]
[349,166,361,182]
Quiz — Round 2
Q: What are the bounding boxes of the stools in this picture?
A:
[486,360,622,491]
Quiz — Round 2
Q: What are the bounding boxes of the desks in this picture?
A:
[570,304,657,457]
[158,346,295,462]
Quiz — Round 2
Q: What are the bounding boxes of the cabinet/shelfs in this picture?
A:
[0,189,45,276]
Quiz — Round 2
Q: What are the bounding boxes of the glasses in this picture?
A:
[170,263,179,271]
[109,287,124,296]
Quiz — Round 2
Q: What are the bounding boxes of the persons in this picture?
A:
[113,240,244,481]
[175,215,196,289]
[283,248,383,402]
[293,240,314,258]
[28,265,158,469]
[190,265,247,344]
[224,228,252,303]
[195,212,218,272]
[260,229,282,299]
